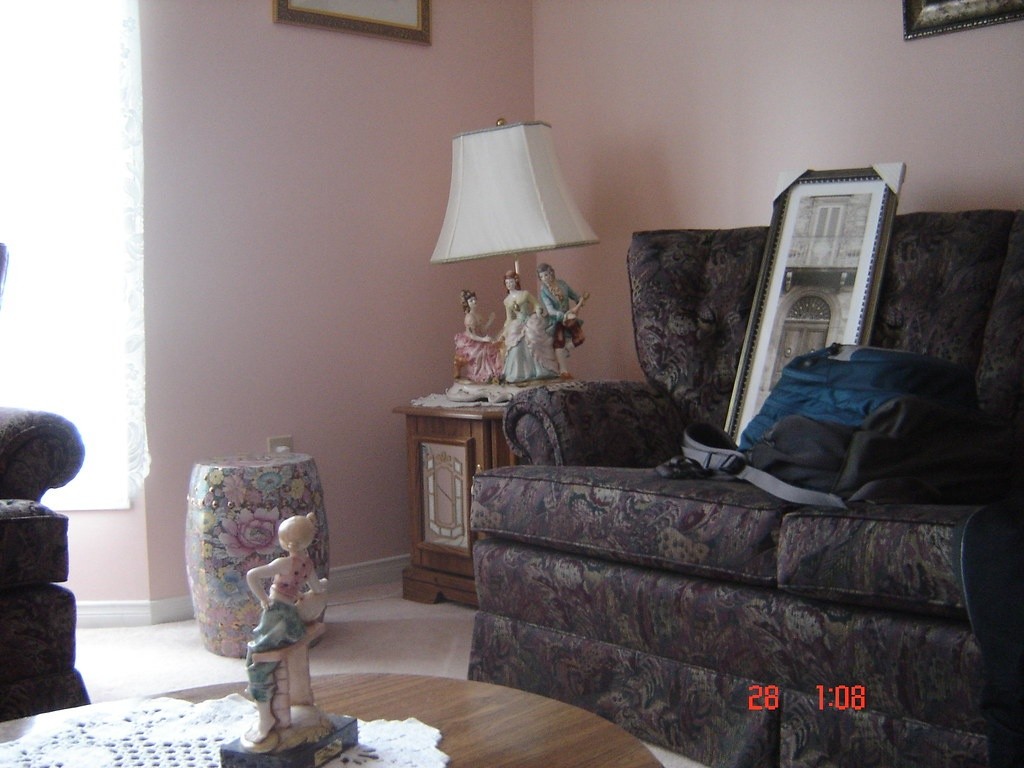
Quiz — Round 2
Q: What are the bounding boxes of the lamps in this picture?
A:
[429,120,601,404]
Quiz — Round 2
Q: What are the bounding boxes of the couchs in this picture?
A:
[0,244,91,726]
[467,209,1024,768]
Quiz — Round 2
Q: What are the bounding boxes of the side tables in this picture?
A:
[391,403,522,609]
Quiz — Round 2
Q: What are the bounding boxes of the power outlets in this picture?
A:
[268,435,293,457]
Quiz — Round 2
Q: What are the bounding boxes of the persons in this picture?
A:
[247,512,318,743]
[503,263,584,383]
[455,289,504,385]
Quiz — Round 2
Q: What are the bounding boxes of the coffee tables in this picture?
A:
[0,672,662,768]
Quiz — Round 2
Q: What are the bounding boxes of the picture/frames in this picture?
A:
[902,0,1024,41]
[723,161,904,448]
[273,0,433,48]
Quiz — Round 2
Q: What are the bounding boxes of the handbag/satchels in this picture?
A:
[654,342,980,510]
